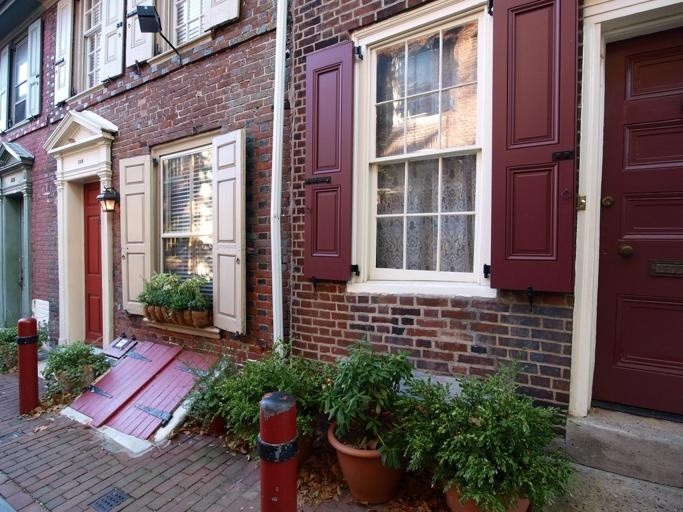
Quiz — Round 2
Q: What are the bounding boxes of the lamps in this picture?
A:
[95,187,119,213]
[135,5,179,62]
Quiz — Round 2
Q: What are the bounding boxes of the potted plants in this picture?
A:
[178,338,576,510]
[38,341,108,396]
[136,269,209,329]
